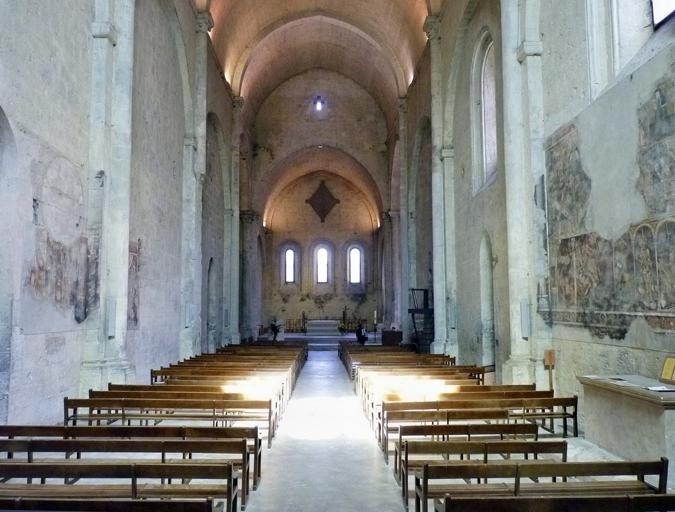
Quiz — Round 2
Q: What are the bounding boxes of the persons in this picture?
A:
[271,321,281,341]
[356,324,368,345]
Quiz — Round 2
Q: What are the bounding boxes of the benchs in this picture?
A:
[0,340,308,512]
[339,343,675,512]
